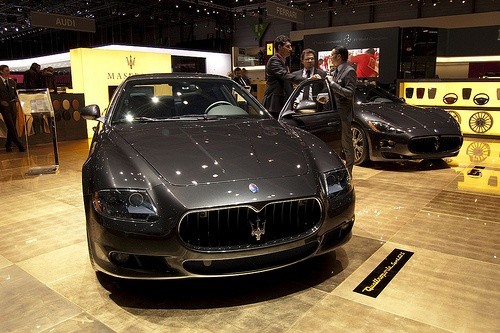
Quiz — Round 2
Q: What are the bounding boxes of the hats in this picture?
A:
[30,63,41,69]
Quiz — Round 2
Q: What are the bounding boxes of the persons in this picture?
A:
[262,35,321,122]
[24,63,58,90]
[289,48,329,113]
[325,45,356,180]
[348,48,376,78]
[0,64,26,153]
[232,68,251,92]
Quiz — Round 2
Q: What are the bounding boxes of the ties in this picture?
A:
[5,79,9,91]
[239,78,244,84]
[303,69,311,100]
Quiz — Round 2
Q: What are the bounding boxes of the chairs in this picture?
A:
[129,92,218,118]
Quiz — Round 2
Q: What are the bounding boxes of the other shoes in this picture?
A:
[5,143,12,152]
[19,145,25,152]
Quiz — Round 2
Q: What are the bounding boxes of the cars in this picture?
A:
[80,72,356,296]
[348,82,463,165]
